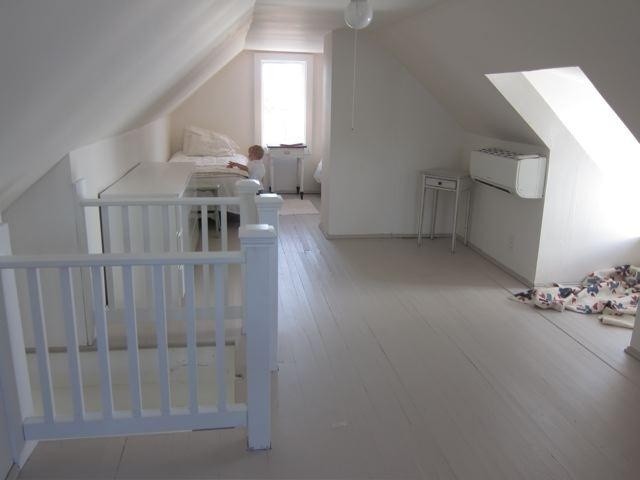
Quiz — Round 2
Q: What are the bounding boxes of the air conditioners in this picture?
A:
[469,148,547,199]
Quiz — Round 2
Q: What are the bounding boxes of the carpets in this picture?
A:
[278,199,319,215]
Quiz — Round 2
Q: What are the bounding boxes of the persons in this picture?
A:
[227,145,265,194]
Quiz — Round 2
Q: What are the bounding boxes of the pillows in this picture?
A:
[183,126,238,156]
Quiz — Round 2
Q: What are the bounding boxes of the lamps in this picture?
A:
[343,1,374,31]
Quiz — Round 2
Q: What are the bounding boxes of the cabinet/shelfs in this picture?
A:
[98,161,195,313]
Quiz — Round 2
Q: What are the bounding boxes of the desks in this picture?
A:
[266,144,306,200]
[417,167,473,255]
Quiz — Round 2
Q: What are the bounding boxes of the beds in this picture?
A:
[167,151,248,215]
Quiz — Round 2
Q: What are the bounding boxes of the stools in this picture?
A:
[195,182,221,238]
